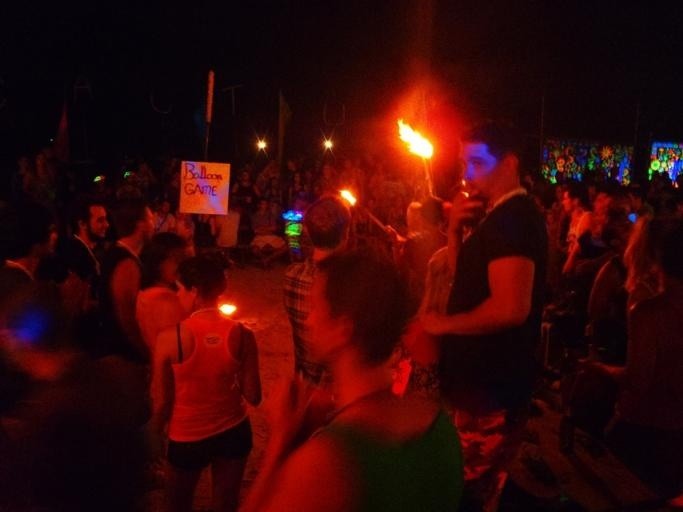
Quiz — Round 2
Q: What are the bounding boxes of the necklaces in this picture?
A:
[116,240,146,269]
[192,306,218,317]
[73,234,100,275]
[484,188,526,213]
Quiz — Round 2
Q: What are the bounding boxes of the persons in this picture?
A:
[1,115,683,512]
